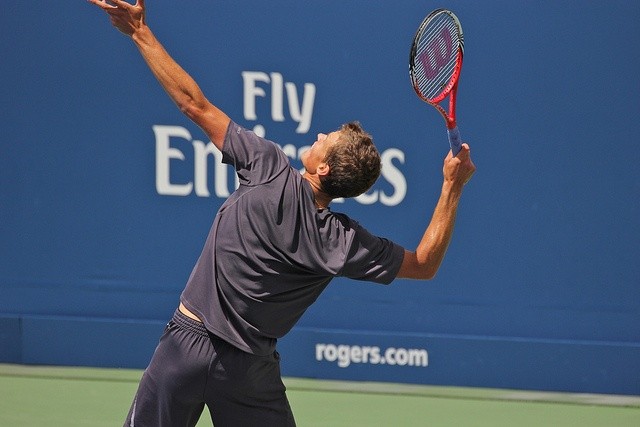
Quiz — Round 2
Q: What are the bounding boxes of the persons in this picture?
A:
[94,1,476,427]
